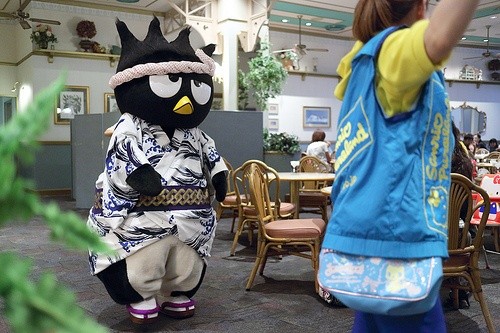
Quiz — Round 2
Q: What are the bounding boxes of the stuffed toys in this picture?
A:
[88,11,232,328]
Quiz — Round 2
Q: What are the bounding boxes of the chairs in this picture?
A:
[442,173,497,333]
[471,174,500,269]
[219,156,328,292]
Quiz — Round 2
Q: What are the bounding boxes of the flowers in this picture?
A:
[77,19,97,41]
[30,25,57,43]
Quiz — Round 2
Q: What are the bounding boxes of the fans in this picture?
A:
[462,25,500,62]
[273,15,328,55]
[0,0,60,30]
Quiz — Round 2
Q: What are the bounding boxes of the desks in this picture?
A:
[263,171,335,219]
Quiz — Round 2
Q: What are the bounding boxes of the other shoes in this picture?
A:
[458,289,470,308]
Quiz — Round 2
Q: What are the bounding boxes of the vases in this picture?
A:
[79,40,92,52]
[39,42,48,49]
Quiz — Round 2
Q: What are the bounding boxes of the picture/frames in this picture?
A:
[268,119,279,131]
[104,93,120,113]
[54,85,90,125]
[303,106,332,128]
[268,103,279,115]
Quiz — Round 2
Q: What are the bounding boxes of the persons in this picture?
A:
[318,0,479,333]
[308,130,500,308]
